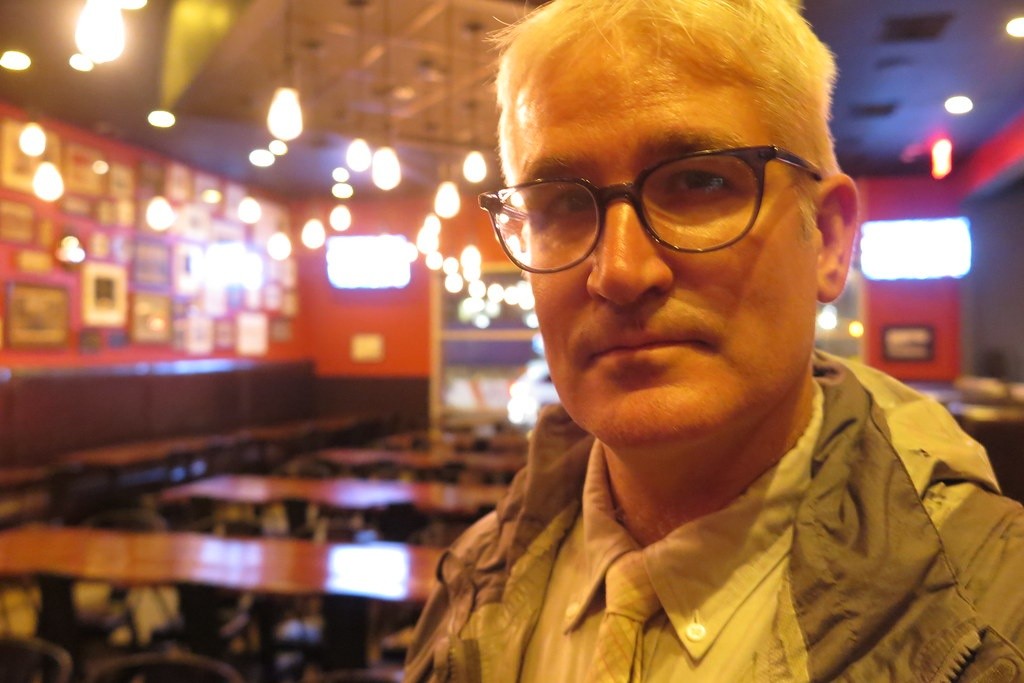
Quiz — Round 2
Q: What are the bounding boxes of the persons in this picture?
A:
[402,1,1024,682]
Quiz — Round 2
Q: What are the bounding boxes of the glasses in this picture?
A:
[477,144,823,274]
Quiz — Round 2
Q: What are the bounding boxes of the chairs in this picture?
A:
[0,410,527,683]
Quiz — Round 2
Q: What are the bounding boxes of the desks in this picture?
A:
[316,448,525,543]
[0,523,450,683]
[386,431,524,486]
[247,417,360,479]
[153,471,509,652]
[55,432,251,528]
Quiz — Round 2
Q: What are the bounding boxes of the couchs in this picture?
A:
[0,356,356,532]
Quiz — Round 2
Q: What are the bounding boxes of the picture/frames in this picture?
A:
[133,235,173,291]
[128,289,174,345]
[81,260,127,327]
[4,279,70,349]
[881,323,936,361]
[0,116,59,199]
